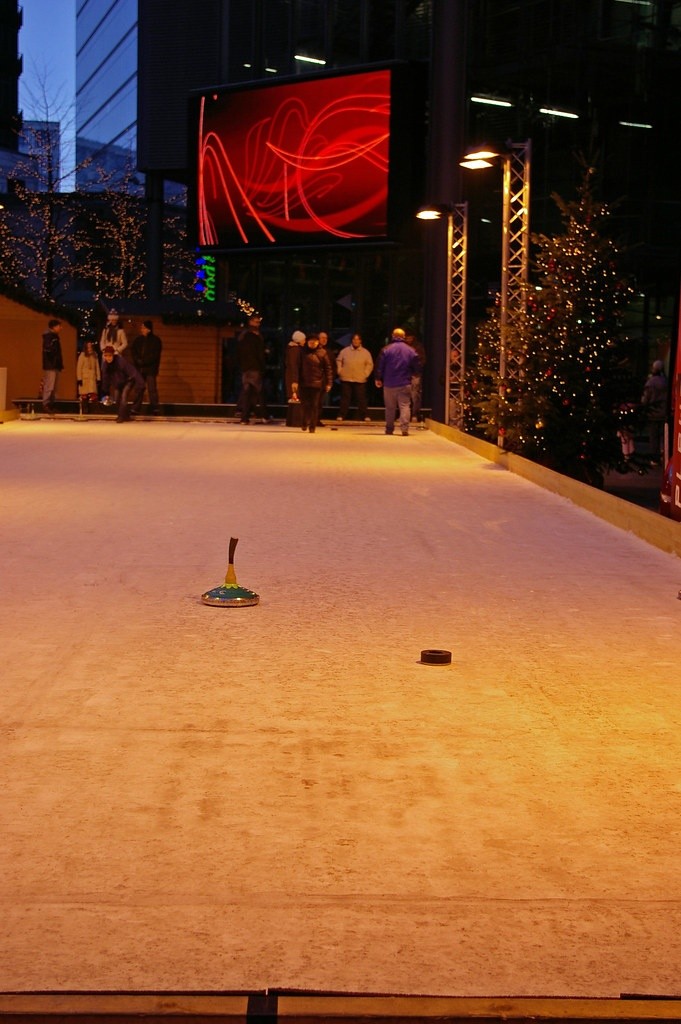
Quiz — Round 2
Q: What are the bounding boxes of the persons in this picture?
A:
[42,309,161,423]
[238,315,434,437]
[640,361,670,468]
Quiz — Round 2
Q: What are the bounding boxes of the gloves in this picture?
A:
[97,381,100,384]
[77,381,82,386]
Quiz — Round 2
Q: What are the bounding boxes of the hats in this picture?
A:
[144,321,152,329]
[292,331,306,342]
[105,346,114,354]
[108,308,119,319]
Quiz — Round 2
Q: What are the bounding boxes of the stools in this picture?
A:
[646,418,664,462]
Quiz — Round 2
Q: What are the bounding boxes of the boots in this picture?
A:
[82,398,88,414]
[91,398,104,413]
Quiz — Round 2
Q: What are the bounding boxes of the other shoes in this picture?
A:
[154,412,160,416]
[117,416,129,423]
[241,421,255,425]
[386,431,392,434]
[337,417,342,420]
[316,422,325,426]
[402,432,408,436]
[310,429,314,432]
[43,409,54,413]
[263,416,272,423]
[128,412,139,415]
[365,418,371,421]
[302,423,306,430]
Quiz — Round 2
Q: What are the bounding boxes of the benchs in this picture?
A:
[11,397,432,422]
